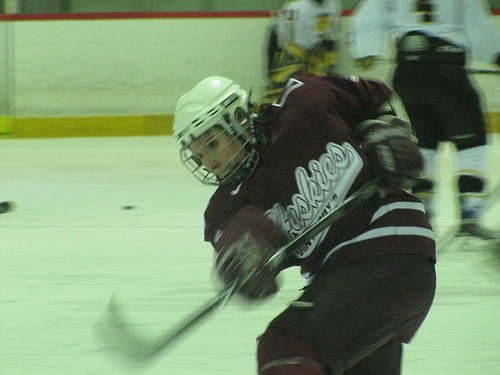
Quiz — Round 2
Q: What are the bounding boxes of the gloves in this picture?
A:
[214,202,292,300]
[358,114,426,190]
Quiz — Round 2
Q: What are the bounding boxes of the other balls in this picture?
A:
[1,202,9,215]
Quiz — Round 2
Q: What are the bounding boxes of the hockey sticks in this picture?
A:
[429,141,461,256]
[96,181,379,369]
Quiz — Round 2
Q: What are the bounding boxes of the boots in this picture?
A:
[459,190,496,241]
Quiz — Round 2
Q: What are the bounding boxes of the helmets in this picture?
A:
[172,75,260,186]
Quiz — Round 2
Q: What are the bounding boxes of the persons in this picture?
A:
[267,0,499,238]
[172,73,437,375]
